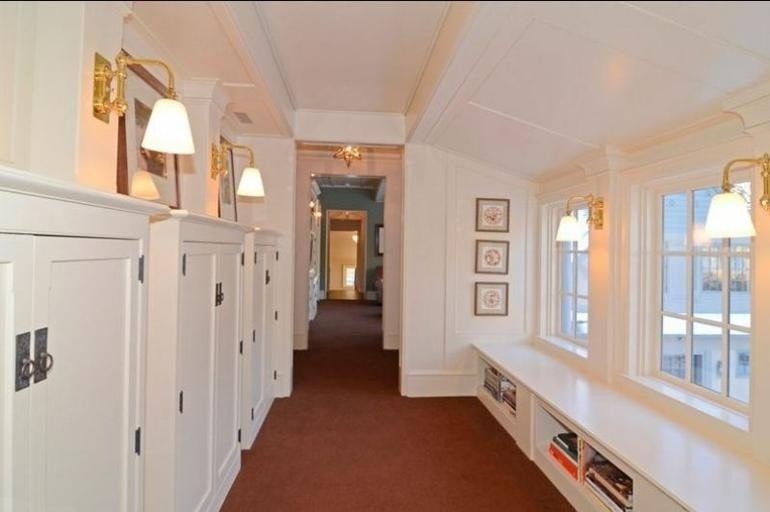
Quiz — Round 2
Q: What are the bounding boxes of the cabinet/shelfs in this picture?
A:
[149,199,256,511]
[0,167,168,510]
[237,220,289,446]
[469,337,769,512]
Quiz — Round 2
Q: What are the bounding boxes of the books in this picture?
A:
[546,431,633,511]
[481,365,516,415]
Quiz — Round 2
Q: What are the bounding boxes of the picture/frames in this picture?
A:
[116,48,188,209]
[375,224,384,257]
[475,195,510,234]
[474,238,510,275]
[473,281,509,317]
[215,134,243,220]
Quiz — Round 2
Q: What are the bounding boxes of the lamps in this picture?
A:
[210,141,265,198]
[92,49,196,156]
[554,191,604,243]
[703,153,770,242]
